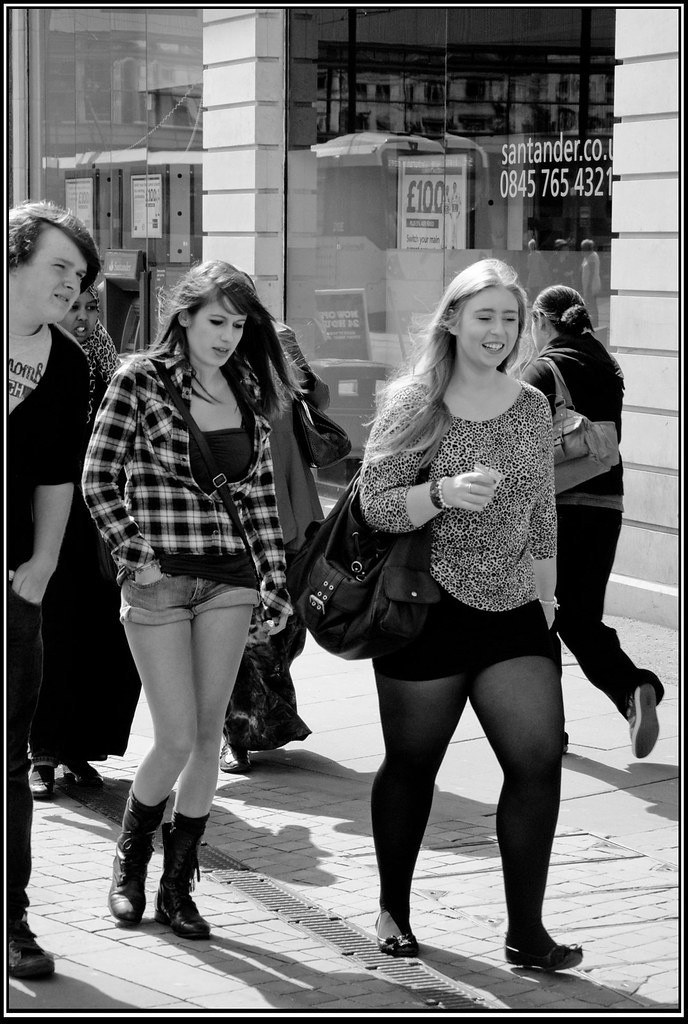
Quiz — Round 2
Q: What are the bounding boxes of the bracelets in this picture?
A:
[539,596,560,610]
[429,480,445,510]
[438,476,452,509]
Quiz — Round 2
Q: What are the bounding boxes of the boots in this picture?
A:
[155,807,213,937]
[108,782,169,924]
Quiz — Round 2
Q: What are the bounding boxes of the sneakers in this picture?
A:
[217,741,254,774]
[628,685,659,758]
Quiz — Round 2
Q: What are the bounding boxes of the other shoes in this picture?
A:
[27,765,55,798]
[374,917,421,960]
[63,757,104,786]
[506,935,582,973]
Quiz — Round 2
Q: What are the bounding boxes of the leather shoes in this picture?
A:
[7,922,55,980]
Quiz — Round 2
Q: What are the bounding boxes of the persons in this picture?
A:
[27,285,144,800]
[525,238,547,303]
[355,257,586,972]
[1,198,100,980]
[521,284,665,760]
[581,238,602,328]
[214,324,331,771]
[549,240,574,288]
[83,258,293,945]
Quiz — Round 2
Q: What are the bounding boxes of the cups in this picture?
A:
[473,460,507,508]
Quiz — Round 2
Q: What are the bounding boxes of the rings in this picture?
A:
[469,483,472,493]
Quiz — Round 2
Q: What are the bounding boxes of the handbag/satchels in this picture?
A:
[221,615,312,750]
[292,392,351,467]
[289,455,440,663]
[536,357,620,499]
